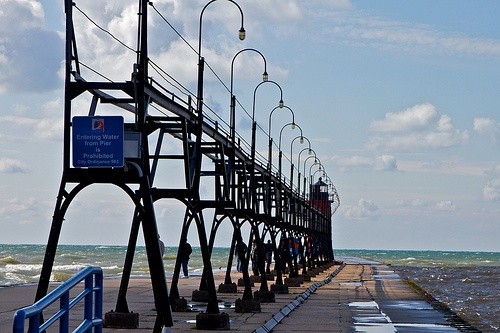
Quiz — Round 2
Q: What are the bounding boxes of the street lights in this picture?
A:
[302,156,341,217]
[196,0,246,120]
[229,49,268,145]
[268,105,295,171]
[277,123,304,181]
[251,80,284,162]
[298,148,317,197]
[290,135,312,191]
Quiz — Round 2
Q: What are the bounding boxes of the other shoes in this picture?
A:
[180,276,189,279]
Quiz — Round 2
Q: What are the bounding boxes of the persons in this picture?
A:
[251,237,274,275]
[158,235,164,257]
[182,239,192,278]
[234,236,247,272]
